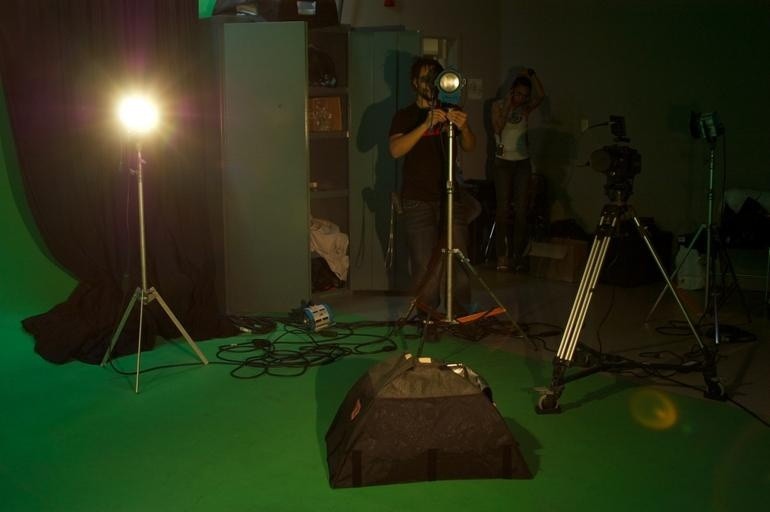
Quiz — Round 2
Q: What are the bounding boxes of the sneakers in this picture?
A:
[417,311,438,341]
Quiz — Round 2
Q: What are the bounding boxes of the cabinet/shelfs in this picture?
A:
[219,19,421,320]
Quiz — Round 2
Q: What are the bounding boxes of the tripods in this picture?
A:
[386,109,537,360]
[99,140,209,393]
[643,148,753,344]
[537,204,723,413]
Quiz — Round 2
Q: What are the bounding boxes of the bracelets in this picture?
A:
[528,68,536,77]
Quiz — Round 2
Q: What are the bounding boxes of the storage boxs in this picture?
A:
[524,236,589,285]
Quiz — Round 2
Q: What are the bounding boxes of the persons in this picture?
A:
[389,57,477,342]
[490,68,546,273]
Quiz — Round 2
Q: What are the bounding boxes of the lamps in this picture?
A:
[401,68,526,357]
